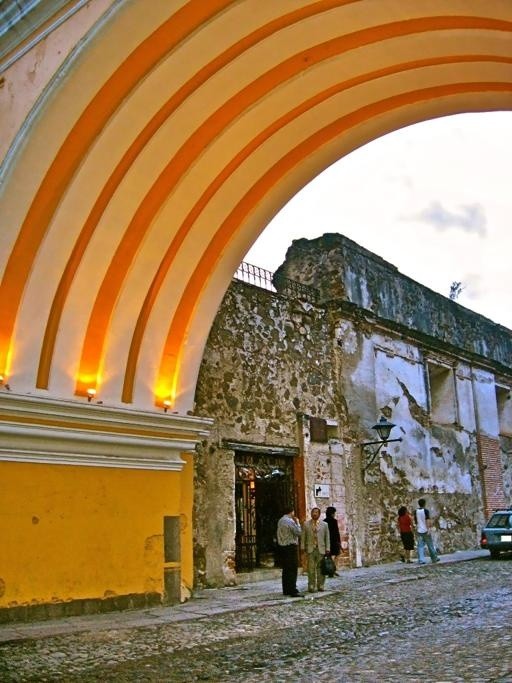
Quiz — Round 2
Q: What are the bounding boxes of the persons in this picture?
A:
[414,498,440,565]
[398,506,415,564]
[301,507,331,592]
[276,506,306,597]
[322,505,342,578]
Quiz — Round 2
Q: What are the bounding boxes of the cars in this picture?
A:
[481,505,512,558]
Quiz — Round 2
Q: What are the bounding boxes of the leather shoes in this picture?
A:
[291,592,304,596]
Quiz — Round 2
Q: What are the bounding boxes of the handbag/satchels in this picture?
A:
[321,554,334,575]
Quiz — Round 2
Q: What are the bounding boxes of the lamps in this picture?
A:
[361,413,402,472]
